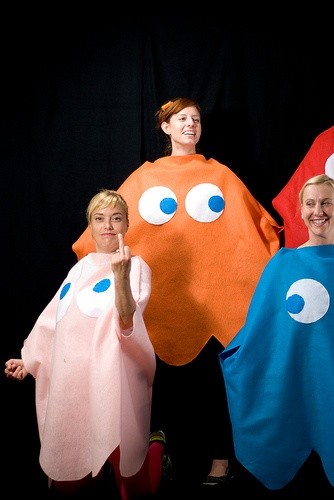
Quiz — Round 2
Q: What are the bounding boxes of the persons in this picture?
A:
[272,127,333,248]
[219,176,334,491]
[70,98,283,487]
[3,188,174,500]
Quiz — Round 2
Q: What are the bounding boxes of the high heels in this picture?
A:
[202,468,234,489]
